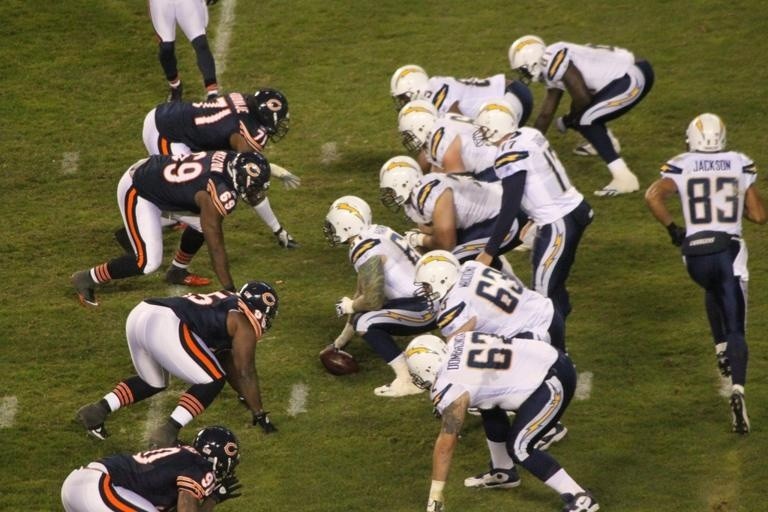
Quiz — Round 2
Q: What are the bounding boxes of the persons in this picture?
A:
[644,111,768,435]
[59,423,244,512]
[75,279,280,451]
[148,0,220,103]
[68,147,272,307]
[322,63,600,511]
[506,33,654,199]
[113,86,302,254]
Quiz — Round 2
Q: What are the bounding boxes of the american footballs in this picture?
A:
[322,350,358,374]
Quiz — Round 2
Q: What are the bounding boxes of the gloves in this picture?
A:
[211,476,243,503]
[275,228,299,248]
[335,296,355,317]
[278,171,302,192]
[667,221,685,245]
[403,231,426,247]
[252,408,277,433]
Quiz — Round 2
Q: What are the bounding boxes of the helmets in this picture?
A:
[324,195,373,244]
[233,151,271,205]
[508,36,545,84]
[390,64,429,111]
[686,112,728,152]
[256,89,290,133]
[398,100,437,152]
[378,156,423,205]
[414,249,462,314]
[405,334,446,392]
[475,104,517,147]
[194,425,241,477]
[240,281,280,334]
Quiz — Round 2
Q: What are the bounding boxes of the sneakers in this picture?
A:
[464,463,521,490]
[149,438,186,449]
[715,351,732,386]
[560,489,600,512]
[166,270,212,286]
[594,177,640,196]
[167,81,182,102]
[373,378,423,397]
[72,404,109,440]
[573,137,620,155]
[728,389,751,434]
[534,423,567,450]
[70,271,100,308]
